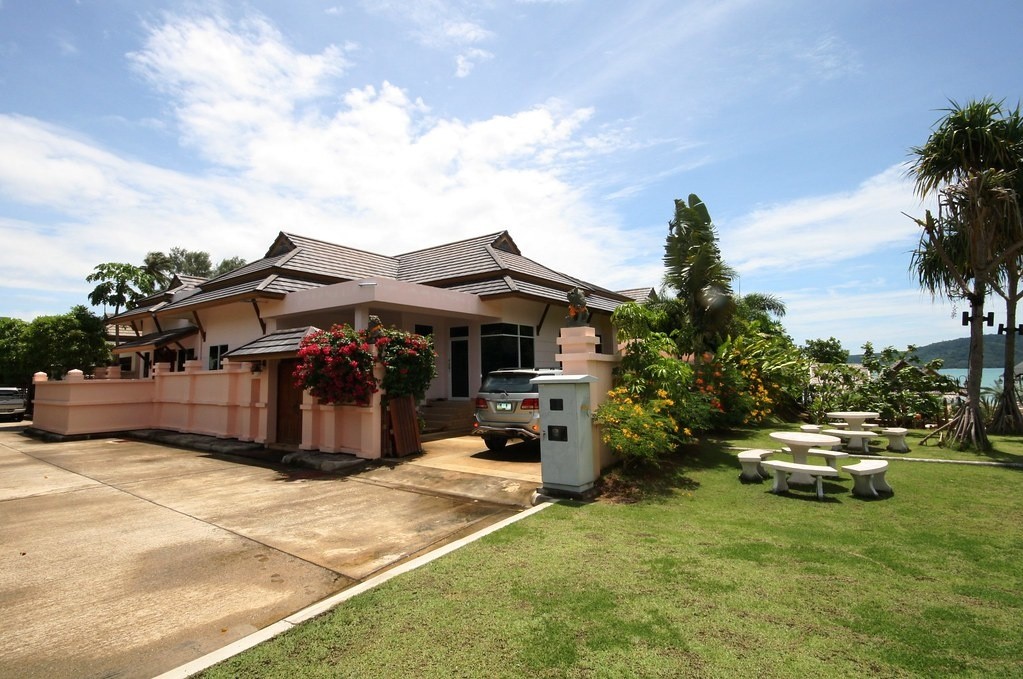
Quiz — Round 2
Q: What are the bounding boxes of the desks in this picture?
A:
[769,431,841,484]
[827,411,879,448]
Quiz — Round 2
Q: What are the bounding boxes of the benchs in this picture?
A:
[737,448,774,485]
[822,429,879,452]
[841,459,892,499]
[780,447,848,470]
[882,427,910,452]
[799,425,823,433]
[828,422,879,440]
[758,460,838,499]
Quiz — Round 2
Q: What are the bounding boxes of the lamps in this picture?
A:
[961,312,972,326]
[998,324,1007,335]
[1015,324,1023,335]
[983,312,995,326]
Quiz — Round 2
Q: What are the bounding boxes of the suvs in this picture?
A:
[0,386,26,421]
[472,367,565,451]
